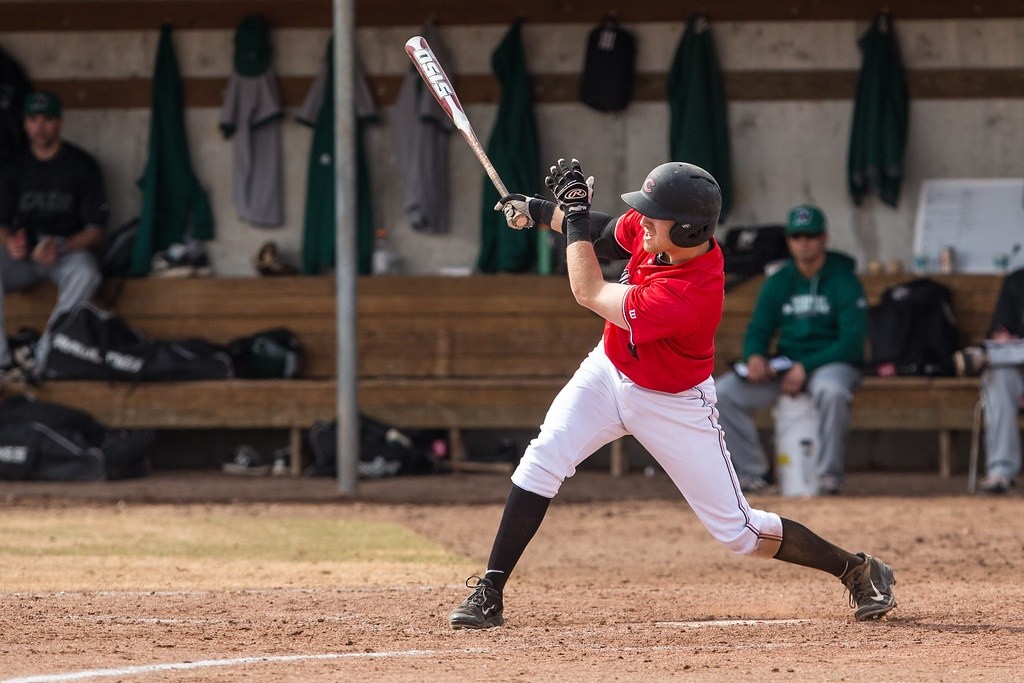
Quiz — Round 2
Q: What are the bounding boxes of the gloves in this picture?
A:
[494,194,548,230]
[545,158,594,223]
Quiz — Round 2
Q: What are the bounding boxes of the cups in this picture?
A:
[868,245,1009,276]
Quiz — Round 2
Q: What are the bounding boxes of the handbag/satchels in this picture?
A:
[154,326,306,379]
[310,409,447,478]
[0,391,151,483]
[872,278,960,379]
[42,302,152,382]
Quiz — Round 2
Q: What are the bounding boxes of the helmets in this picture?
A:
[621,162,722,247]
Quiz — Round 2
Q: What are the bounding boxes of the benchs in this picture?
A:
[0,277,1024,484]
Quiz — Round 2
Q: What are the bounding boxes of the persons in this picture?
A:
[450,158,897,629]
[715,203,867,501]
[953,265,1024,492]
[0,88,110,388]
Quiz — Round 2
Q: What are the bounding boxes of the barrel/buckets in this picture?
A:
[771,404,820,499]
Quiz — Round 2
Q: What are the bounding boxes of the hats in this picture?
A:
[784,204,826,238]
[579,20,642,114]
[234,17,273,76]
[17,91,60,115]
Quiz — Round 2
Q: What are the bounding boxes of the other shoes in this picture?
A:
[818,471,844,494]
[740,476,767,493]
[980,470,1013,491]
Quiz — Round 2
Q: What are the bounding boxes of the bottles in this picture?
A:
[371,228,388,275]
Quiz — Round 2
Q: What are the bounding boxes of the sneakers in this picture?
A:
[842,553,895,619]
[271,455,291,474]
[150,249,192,279]
[449,576,504,631]
[177,249,213,276]
[220,445,271,477]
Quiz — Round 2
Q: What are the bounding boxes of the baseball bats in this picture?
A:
[404,35,530,231]
